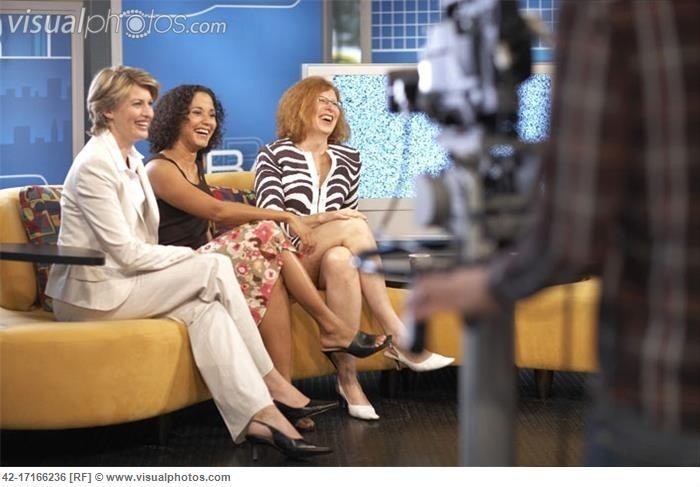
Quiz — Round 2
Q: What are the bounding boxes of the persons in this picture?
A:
[43,63,340,463]
[405,0,700,467]
[253,75,456,419]
[142,84,392,432]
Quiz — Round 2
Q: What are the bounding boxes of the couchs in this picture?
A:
[0,170,604,441]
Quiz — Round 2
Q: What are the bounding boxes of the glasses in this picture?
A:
[317,96,342,109]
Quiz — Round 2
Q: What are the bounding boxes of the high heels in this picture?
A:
[273,397,339,418]
[335,377,380,421]
[321,329,392,369]
[385,342,455,373]
[245,419,333,462]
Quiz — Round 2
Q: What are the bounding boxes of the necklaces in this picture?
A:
[186,174,193,180]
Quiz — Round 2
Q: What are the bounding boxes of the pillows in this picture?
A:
[207,185,258,238]
[20,185,62,313]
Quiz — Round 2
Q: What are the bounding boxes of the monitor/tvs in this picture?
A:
[299,62,558,242]
